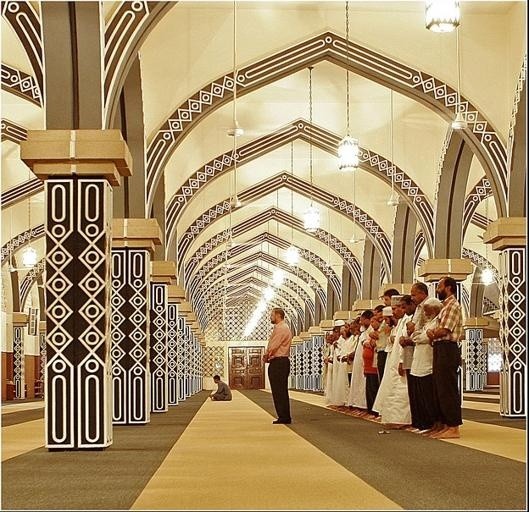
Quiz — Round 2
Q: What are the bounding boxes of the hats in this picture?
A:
[390,294,403,307]
[334,319,346,327]
[382,305,394,317]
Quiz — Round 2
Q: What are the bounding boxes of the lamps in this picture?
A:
[427,1,461,32]
[337,1,360,172]
[349,172,359,244]
[285,139,300,268]
[451,29,467,129]
[230,135,242,208]
[21,169,38,267]
[386,88,400,206]
[226,210,235,248]
[481,198,495,286]
[6,206,16,273]
[227,1,244,136]
[273,188,284,287]
[303,65,321,232]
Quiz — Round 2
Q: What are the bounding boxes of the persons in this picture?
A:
[323,288,406,428]
[426,276,466,438]
[263,308,293,424]
[399,295,417,432]
[209,375,232,401]
[405,301,442,435]
[407,282,442,432]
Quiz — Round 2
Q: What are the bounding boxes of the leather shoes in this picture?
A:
[273,417,291,424]
